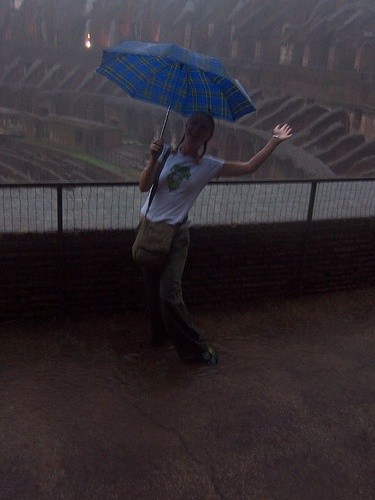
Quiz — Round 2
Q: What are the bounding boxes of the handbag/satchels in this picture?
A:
[132,217,173,267]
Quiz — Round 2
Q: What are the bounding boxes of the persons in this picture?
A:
[132,111,294,366]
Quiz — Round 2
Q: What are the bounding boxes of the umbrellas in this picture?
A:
[96,45,256,140]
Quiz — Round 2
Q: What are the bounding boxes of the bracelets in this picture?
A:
[144,166,153,173]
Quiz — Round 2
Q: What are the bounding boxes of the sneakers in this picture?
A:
[199,342,217,368]
